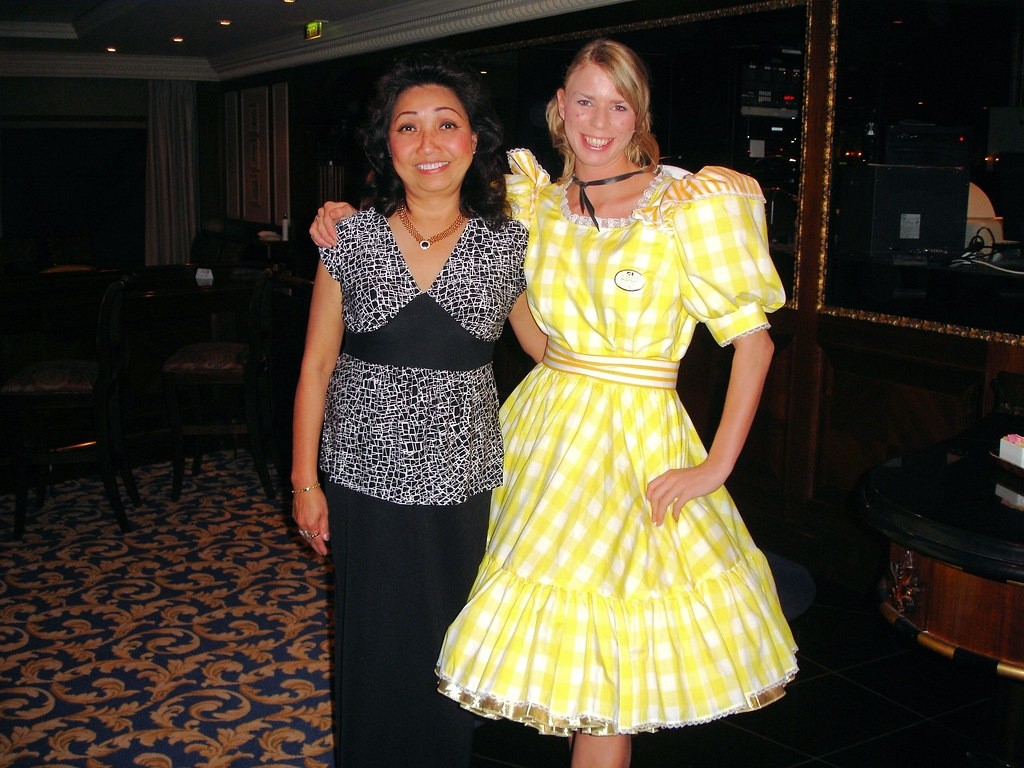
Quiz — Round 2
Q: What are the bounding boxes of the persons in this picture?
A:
[435,39,799,768]
[290,47,547,768]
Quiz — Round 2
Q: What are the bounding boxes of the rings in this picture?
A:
[298,529,311,543]
[307,532,320,539]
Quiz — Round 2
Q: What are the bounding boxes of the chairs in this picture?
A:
[161,267,285,498]
[1,281,140,541]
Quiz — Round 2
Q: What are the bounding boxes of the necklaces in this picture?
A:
[572,168,650,233]
[397,196,465,251]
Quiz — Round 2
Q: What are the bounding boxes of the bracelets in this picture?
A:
[290,482,321,494]
